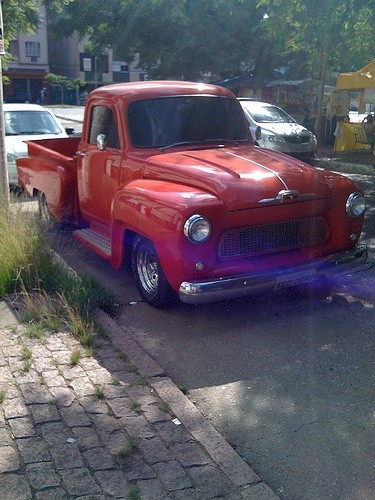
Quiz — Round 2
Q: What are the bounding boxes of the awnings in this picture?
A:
[206,72,321,90]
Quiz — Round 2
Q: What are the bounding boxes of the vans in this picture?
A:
[1,102,68,189]
[233,96,320,164]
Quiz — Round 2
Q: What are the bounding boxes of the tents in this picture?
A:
[332,57,374,154]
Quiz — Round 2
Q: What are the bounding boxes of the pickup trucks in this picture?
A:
[13,80,372,312]
